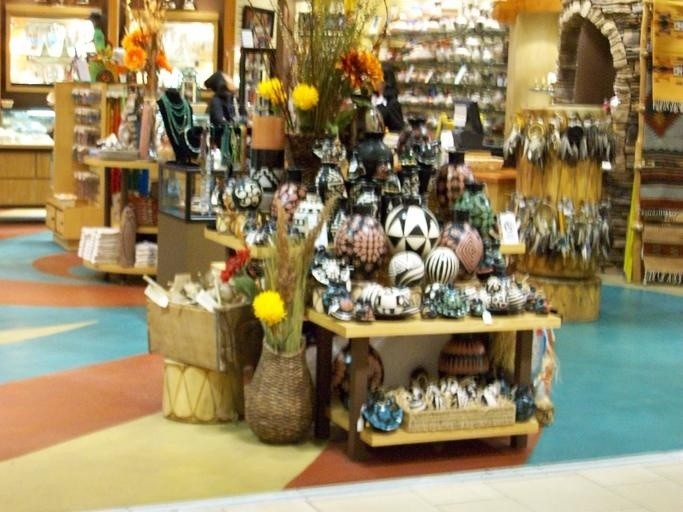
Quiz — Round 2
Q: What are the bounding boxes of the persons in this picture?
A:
[203,70,237,150]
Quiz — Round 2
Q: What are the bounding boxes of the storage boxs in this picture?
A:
[145,295,260,373]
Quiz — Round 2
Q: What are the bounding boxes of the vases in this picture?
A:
[281,133,338,177]
[245,351,315,443]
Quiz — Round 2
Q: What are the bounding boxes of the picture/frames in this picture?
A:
[242,5,274,39]
[240,48,276,118]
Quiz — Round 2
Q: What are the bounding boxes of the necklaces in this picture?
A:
[159,92,206,155]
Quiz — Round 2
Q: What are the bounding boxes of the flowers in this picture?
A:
[240,2,389,160]
[220,194,343,352]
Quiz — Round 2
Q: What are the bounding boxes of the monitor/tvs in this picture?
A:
[453,97,483,134]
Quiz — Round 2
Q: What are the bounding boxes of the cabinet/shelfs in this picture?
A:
[393,0,509,155]
[300,305,562,463]
